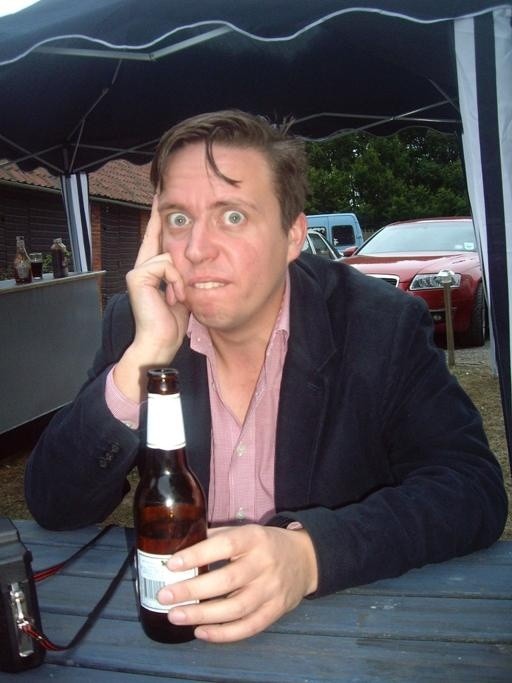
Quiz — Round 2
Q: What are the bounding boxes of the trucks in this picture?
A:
[304,211,366,257]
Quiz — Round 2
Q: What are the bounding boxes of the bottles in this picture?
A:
[13,236,33,285]
[51,238,69,279]
[131,367,208,644]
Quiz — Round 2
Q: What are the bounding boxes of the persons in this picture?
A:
[23,105,510,645]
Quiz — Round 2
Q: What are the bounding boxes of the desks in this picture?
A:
[0,270,108,433]
[1,518,511,683]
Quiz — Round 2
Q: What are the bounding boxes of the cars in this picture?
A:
[299,229,342,261]
[335,214,489,350]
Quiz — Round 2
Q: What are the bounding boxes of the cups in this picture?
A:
[30,253,43,280]
